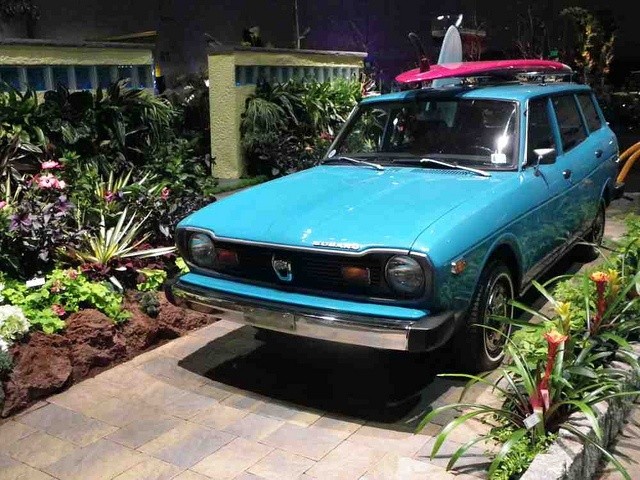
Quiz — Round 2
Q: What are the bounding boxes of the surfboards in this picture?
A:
[424,26,463,126]
[395,32,572,83]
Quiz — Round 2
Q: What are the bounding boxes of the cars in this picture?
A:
[169,83,625,374]
